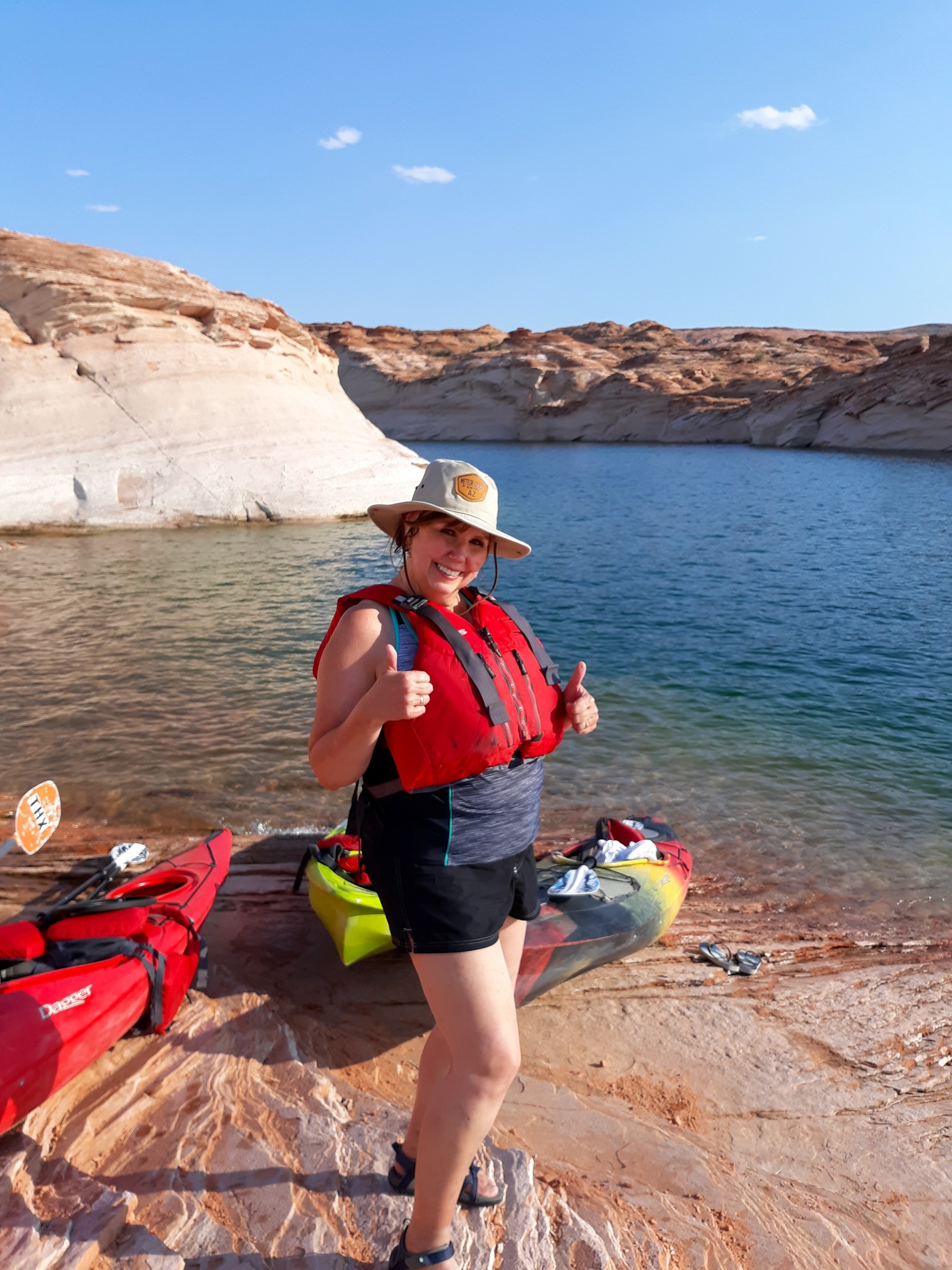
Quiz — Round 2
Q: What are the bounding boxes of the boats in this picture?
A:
[0,827,234,1140]
[302,814,694,1012]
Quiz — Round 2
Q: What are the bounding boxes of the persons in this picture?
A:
[307,458,599,1270]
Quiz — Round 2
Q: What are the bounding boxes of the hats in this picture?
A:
[367,459,532,559]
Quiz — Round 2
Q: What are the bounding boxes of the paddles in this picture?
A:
[546,819,643,898]
[41,842,150,918]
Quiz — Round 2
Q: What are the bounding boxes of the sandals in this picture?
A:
[386,1140,503,1206]
[389,1218,456,1270]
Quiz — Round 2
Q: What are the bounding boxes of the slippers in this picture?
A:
[735,949,762,975]
[699,941,740,972]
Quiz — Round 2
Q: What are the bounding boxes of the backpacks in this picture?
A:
[318,835,374,890]
